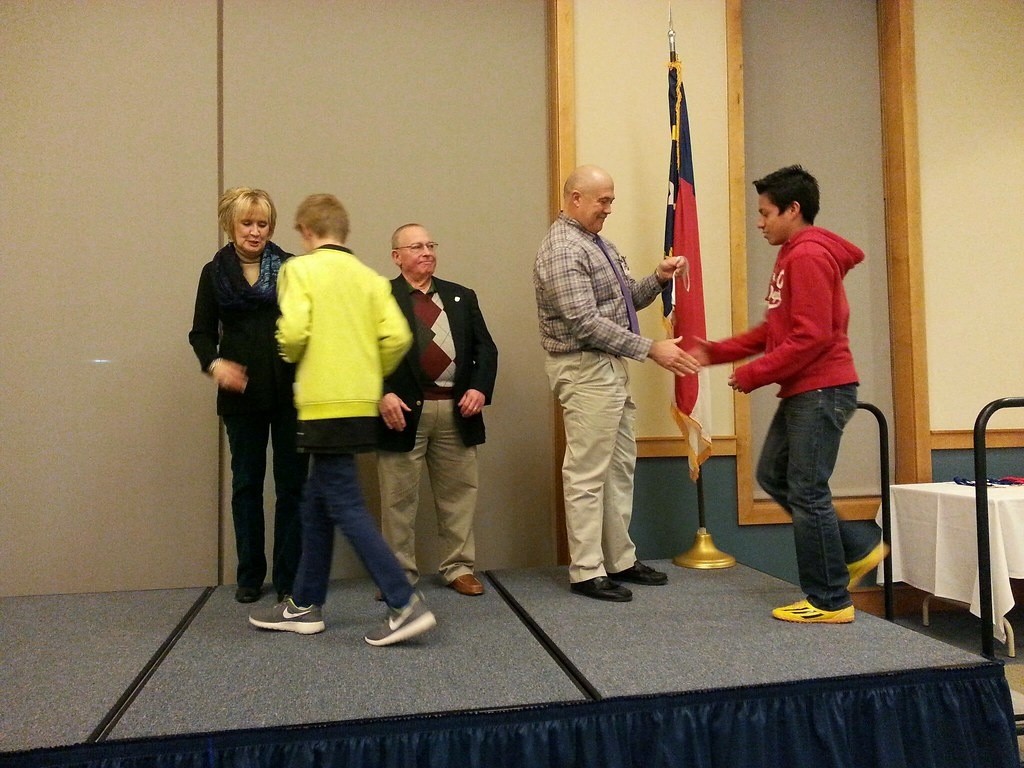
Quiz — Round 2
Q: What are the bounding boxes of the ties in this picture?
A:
[595,233,640,335]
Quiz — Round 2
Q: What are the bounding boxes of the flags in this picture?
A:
[661,55,716,484]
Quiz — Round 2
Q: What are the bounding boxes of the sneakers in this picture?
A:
[846,540,890,591]
[771,597,855,623]
[363,593,437,646]
[248,594,326,635]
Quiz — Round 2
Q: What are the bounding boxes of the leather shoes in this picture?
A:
[449,574,484,595]
[570,576,633,602]
[607,561,668,585]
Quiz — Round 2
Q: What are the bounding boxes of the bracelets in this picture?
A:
[208,358,221,376]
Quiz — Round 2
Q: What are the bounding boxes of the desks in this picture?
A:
[874,480,1024,659]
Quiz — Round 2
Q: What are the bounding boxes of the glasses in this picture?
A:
[392,242,438,253]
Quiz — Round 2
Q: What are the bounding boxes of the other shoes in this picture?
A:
[235,586,262,603]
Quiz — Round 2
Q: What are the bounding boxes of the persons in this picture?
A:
[687,167,889,623]
[535,165,703,602]
[377,223,498,601]
[189,187,304,603]
[249,195,437,645]
